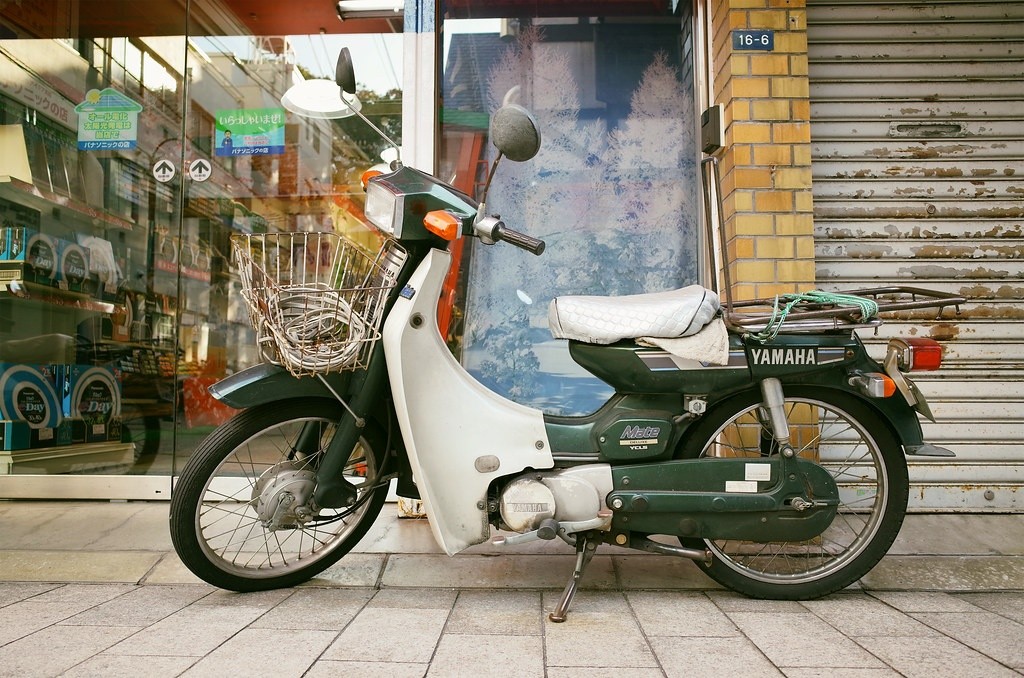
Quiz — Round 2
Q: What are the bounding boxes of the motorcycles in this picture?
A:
[165,51,967,630]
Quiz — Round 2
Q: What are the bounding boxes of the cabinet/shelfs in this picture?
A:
[0,102,142,471]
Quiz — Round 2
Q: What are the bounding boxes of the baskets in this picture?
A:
[230,231,398,380]
